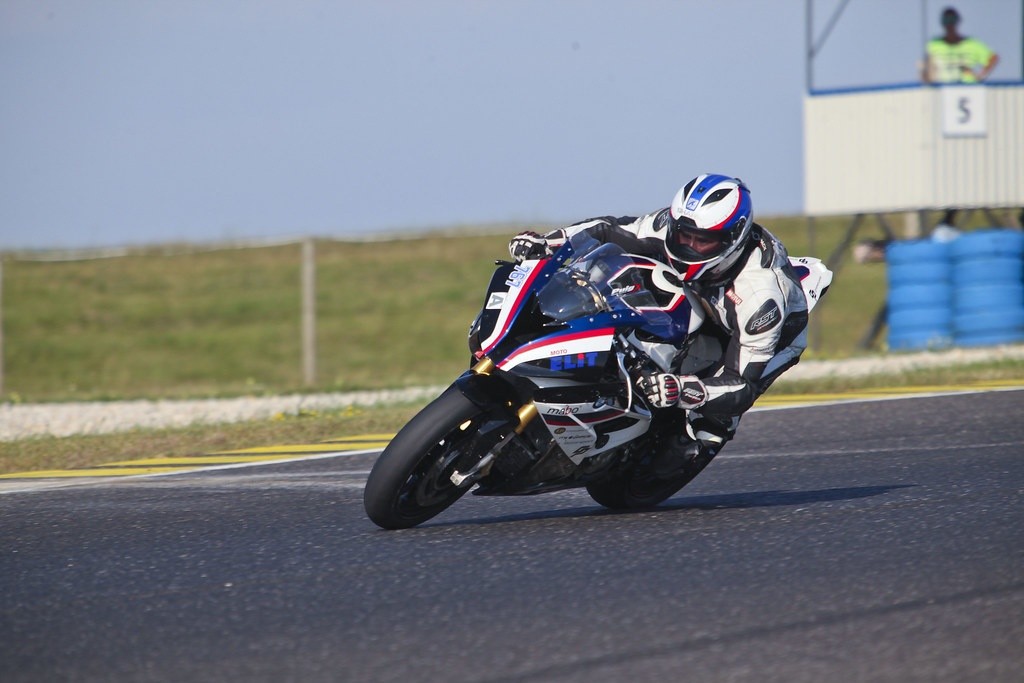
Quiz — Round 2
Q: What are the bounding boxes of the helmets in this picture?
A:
[664,173,752,283]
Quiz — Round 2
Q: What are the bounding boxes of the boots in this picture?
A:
[628,419,723,501]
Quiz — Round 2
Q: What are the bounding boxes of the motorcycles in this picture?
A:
[363,227,836,531]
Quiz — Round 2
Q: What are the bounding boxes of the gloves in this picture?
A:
[637,374,710,410]
[508,227,567,263]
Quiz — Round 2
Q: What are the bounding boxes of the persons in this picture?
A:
[922,6,999,85]
[509,172,809,469]
[930,209,962,242]
